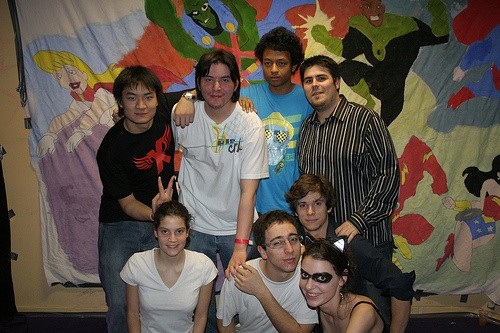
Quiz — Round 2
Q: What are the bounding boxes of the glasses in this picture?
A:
[262,235,303,249]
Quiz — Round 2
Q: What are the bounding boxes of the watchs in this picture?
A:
[182,92,196,101]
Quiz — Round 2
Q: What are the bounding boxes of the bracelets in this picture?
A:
[234,238,253,246]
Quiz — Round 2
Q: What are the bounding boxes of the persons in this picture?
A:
[297,54,401,261]
[299,233,389,333]
[216,210,319,333]
[96,64,197,333]
[171,49,269,281]
[238,26,316,215]
[119,201,219,332]
[285,174,416,333]
